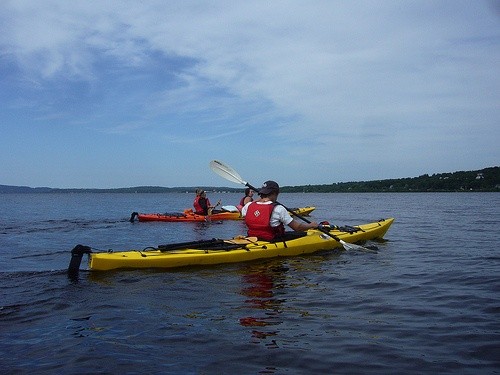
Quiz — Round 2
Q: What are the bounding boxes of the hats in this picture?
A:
[195,189,204,196]
[257,181,279,196]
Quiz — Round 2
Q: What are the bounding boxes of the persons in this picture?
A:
[241,181,320,244]
[240,188,254,207]
[193,188,211,215]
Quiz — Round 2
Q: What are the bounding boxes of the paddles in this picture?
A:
[210,159,374,256]
[207,199,222,216]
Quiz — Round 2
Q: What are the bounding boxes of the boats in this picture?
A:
[129,206,316,222]
[67,217,395,278]
